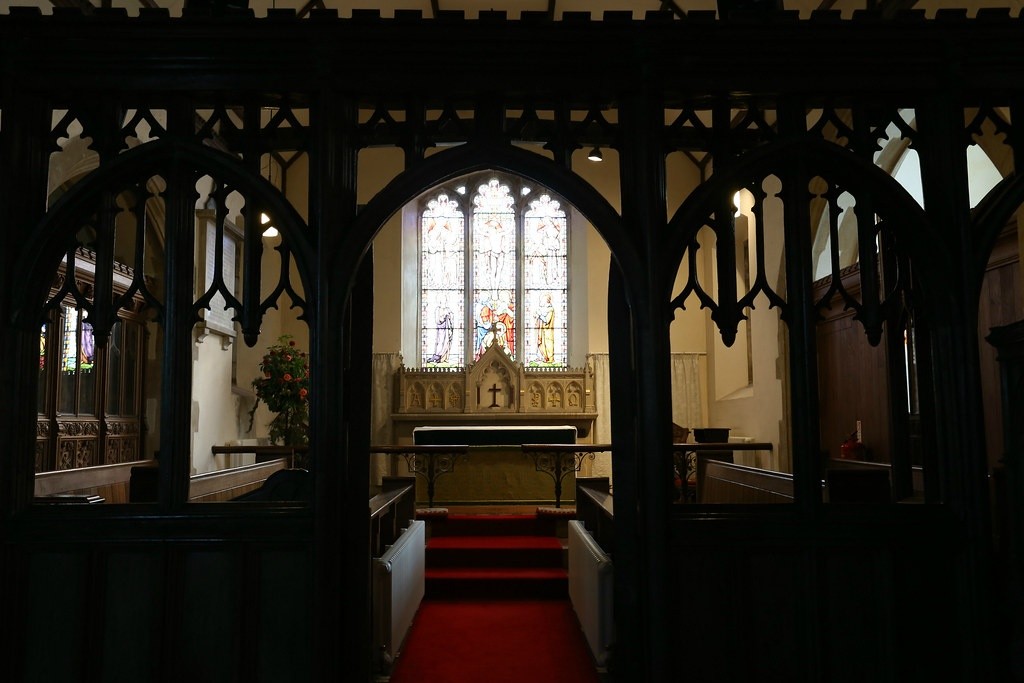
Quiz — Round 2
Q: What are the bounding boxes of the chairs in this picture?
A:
[672,424,691,503]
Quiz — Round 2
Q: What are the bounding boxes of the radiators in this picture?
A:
[372,519,426,665]
[568,520,614,667]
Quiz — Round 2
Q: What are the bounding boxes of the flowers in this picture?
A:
[245,334,310,468]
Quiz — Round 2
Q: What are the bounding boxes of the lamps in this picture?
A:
[588,146,603,161]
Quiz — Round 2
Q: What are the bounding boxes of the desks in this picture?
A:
[369,475,415,558]
[412,425,578,508]
[576,478,614,562]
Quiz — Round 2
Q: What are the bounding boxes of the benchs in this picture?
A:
[34,457,288,503]
[699,458,926,504]
